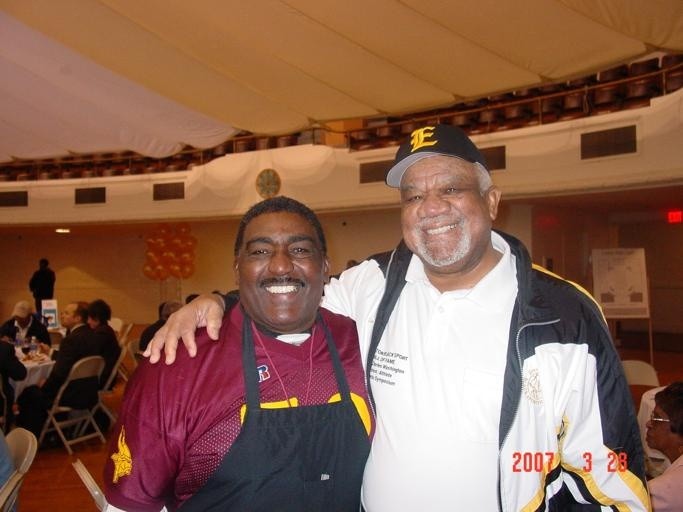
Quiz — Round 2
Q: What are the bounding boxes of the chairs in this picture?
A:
[0,427,38,512]
[621,360,659,388]
[49,331,63,345]
[126,337,140,369]
[117,322,135,347]
[76,346,127,438]
[38,355,107,455]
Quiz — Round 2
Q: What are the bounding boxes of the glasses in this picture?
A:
[648,408,671,426]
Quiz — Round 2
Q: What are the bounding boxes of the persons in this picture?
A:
[0,326,29,435]
[140,124,654,510]
[14,300,101,450]
[0,430,20,512]
[640,380,682,512]
[28,257,56,312]
[137,289,226,356]
[85,298,120,391]
[0,299,51,348]
[96,195,376,511]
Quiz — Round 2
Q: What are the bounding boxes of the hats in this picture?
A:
[386,125,483,189]
[10,300,34,319]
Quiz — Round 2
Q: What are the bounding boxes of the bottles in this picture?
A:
[30,336,37,354]
[15,332,22,351]
